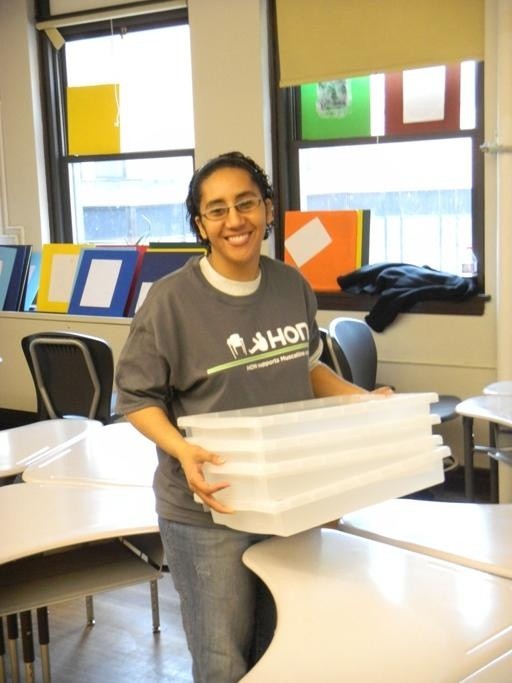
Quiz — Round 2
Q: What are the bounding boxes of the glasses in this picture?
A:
[198,192,267,220]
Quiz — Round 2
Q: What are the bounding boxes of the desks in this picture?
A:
[454,379,512,503]
[233,527,512,683]
[334,497,512,580]
[1,415,166,682]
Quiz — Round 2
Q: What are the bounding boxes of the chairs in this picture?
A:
[22,328,128,426]
[327,318,464,469]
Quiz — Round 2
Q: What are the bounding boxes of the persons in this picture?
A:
[109,150,394,681]
